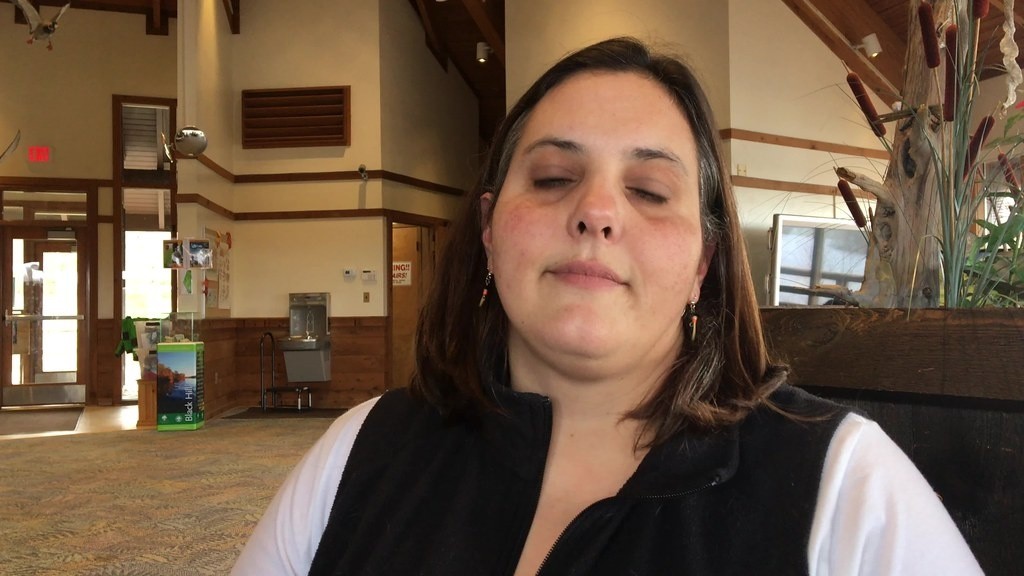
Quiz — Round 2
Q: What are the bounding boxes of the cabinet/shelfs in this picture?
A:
[289,292,331,337]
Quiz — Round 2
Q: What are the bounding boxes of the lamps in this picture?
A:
[852,32,884,62]
[475,42,492,63]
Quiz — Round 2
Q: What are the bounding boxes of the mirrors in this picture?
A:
[768,213,872,309]
[175,126,208,157]
[161,134,173,164]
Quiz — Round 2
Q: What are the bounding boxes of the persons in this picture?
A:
[228,37,985,576]
